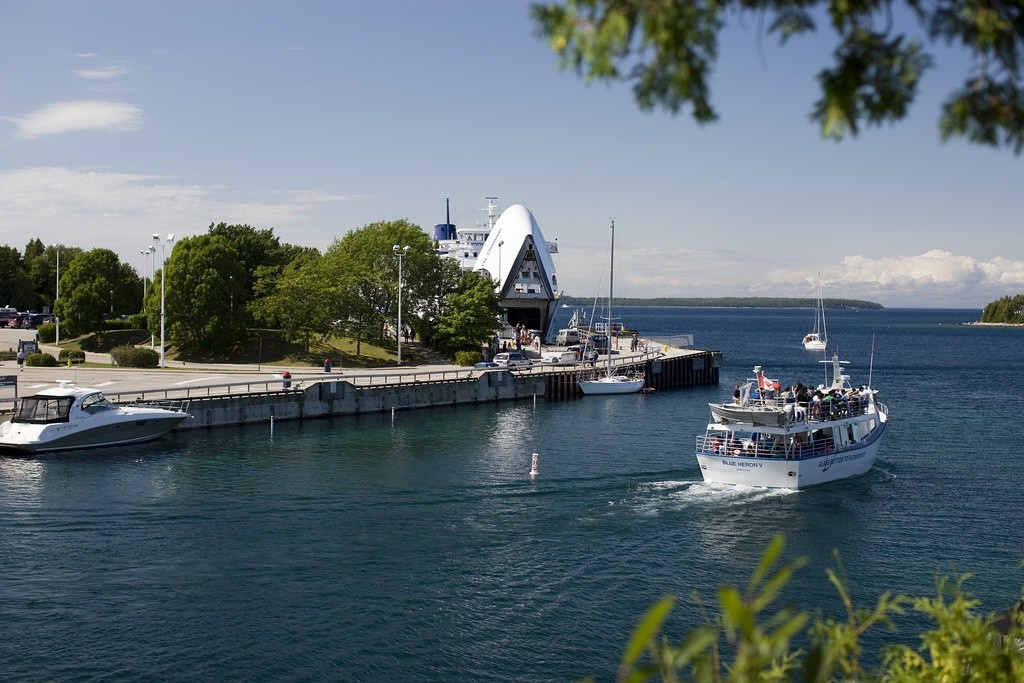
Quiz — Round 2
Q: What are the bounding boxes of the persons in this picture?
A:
[534,335,539,349]
[712,434,756,457]
[405,329,408,343]
[781,383,866,422]
[17,349,24,371]
[492,336,500,348]
[752,388,773,404]
[502,341,506,352]
[516,323,535,345]
[631,334,637,352]
[516,337,521,348]
[508,342,511,351]
[589,348,598,366]
[613,324,617,331]
[733,384,740,404]
[813,429,823,440]
[410,329,415,342]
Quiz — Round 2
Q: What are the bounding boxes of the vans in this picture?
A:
[587,334,610,348]
[555,329,580,346]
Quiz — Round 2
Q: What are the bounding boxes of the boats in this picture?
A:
[692,350,889,489]
[0,380,191,451]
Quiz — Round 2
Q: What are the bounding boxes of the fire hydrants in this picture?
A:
[67,358,72,369]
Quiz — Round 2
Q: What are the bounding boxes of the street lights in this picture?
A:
[140,250,151,314]
[393,245,411,367]
[148,246,156,280]
[53,242,65,346]
[152,234,175,367]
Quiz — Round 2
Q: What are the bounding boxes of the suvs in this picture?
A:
[493,352,534,371]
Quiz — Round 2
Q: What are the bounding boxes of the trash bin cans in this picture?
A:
[324,359,331,372]
[283,372,291,388]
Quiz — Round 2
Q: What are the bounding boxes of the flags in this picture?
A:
[757,374,779,391]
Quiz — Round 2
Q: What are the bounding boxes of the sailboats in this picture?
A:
[576,217,646,395]
[800,271,830,352]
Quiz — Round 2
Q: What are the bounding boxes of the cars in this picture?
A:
[0,307,57,329]
[475,362,500,369]
